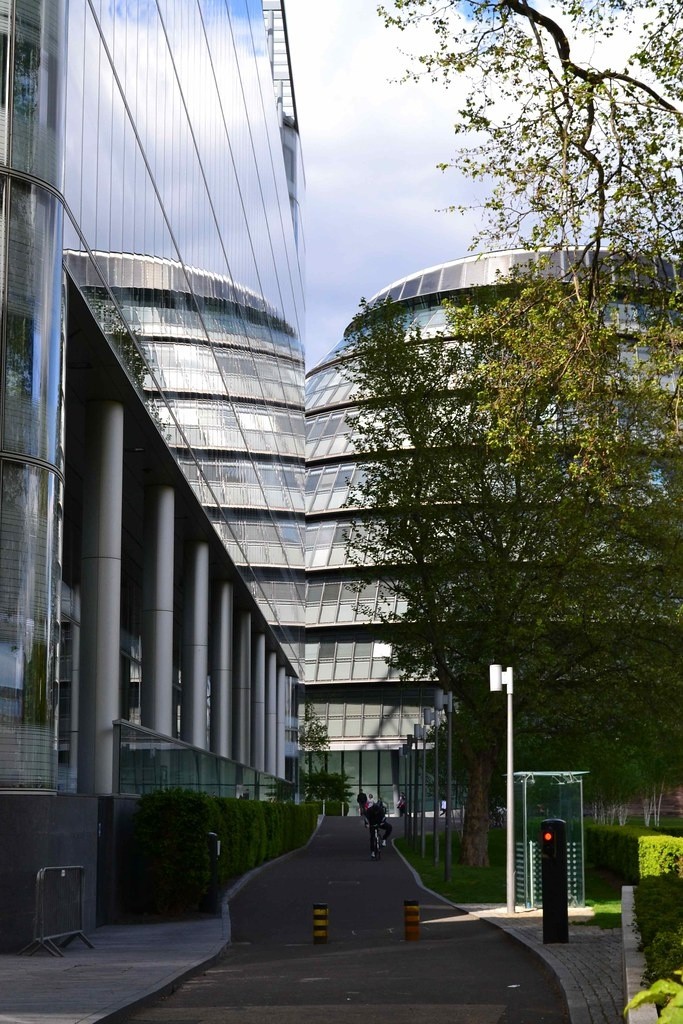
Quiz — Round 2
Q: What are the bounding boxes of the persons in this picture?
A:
[492,808,503,827]
[365,805,392,858]
[357,789,374,816]
[396,792,409,817]
[537,801,545,816]
[243,789,249,799]
[440,800,446,816]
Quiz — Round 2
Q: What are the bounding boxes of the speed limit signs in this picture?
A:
[540,818,569,943]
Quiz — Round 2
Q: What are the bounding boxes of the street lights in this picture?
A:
[399,746,413,836]
[433,688,452,882]
[406,733,417,842]
[423,707,439,861]
[488,662,516,910]
[414,724,426,856]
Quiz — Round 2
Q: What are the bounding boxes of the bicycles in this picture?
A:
[373,825,381,861]
